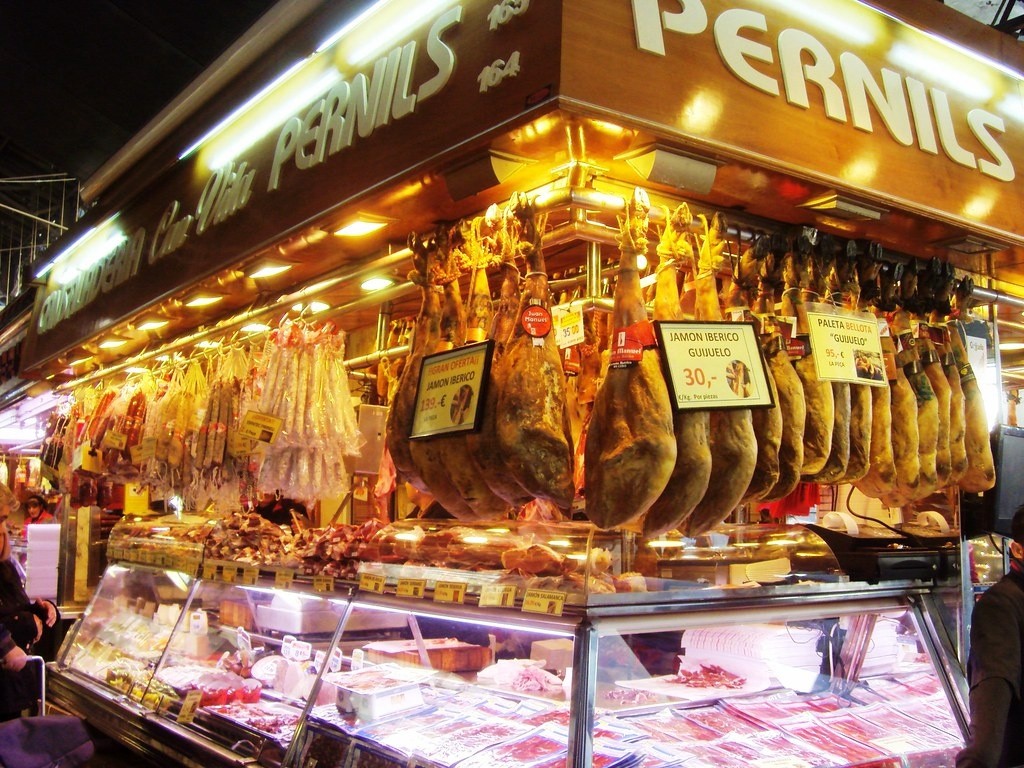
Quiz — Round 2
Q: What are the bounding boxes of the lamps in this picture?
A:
[436,148,540,203]
[131,312,179,330]
[95,334,135,348]
[795,194,890,223]
[240,255,304,278]
[325,211,402,236]
[41,364,71,381]
[178,286,233,306]
[614,142,728,195]
[65,347,99,366]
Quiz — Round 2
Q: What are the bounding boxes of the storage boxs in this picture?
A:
[325,660,439,718]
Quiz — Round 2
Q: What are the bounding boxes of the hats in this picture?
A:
[30,494,48,508]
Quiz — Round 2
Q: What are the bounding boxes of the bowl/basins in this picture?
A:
[648,540,687,558]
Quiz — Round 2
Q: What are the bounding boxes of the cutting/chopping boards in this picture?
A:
[368,637,492,672]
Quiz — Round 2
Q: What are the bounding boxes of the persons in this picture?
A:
[955,507,1024,768]
[18,494,53,563]
[251,479,309,579]
[0,483,61,723]
[404,479,490,646]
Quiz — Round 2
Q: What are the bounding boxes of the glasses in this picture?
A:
[22,500,42,511]
